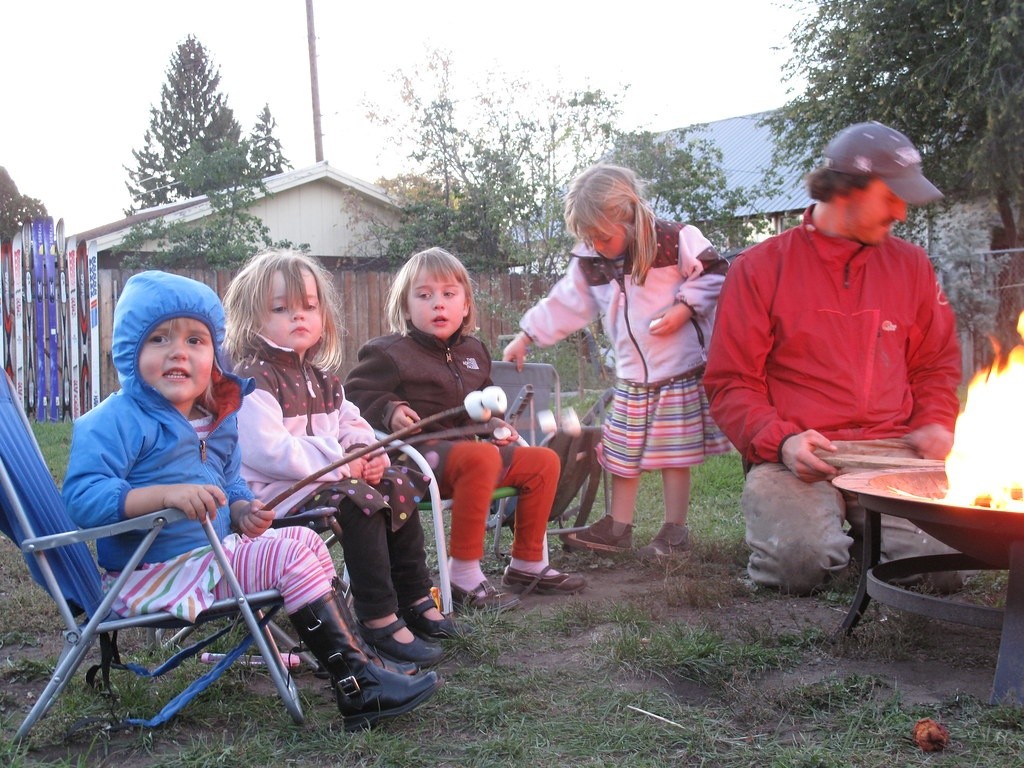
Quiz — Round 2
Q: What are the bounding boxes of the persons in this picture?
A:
[700,122,979,597]
[223,251,475,670]
[343,247,591,618]
[503,164,732,566]
[61,270,446,731]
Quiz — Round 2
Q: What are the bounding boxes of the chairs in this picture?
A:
[0,365,304,746]
[488,360,618,559]
[370,427,550,615]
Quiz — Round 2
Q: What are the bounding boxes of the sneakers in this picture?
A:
[559,512,632,553]
[639,522,692,560]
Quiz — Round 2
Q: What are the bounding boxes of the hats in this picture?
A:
[823,120,945,202]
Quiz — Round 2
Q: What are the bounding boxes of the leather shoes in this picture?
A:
[396,593,472,644]
[353,612,440,664]
[449,574,520,612]
[501,563,585,599]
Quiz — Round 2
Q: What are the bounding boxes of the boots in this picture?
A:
[286,578,438,735]
